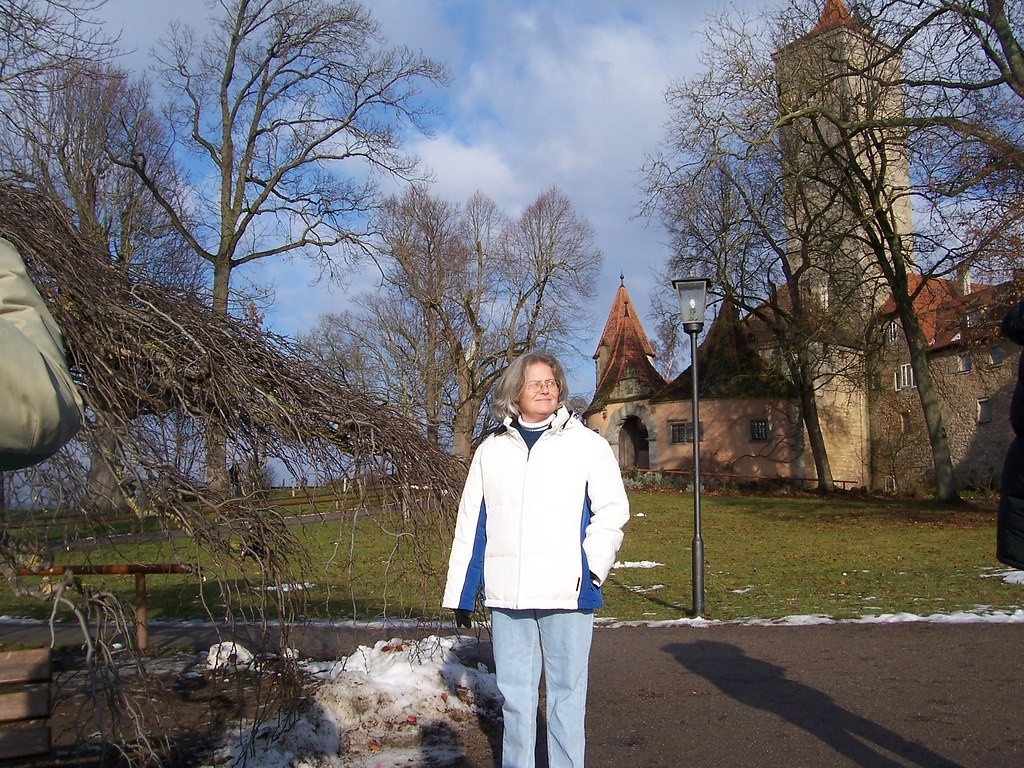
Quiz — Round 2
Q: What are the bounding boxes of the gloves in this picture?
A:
[454,609,473,629]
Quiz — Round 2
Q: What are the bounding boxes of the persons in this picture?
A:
[442,351,630,768]
[994,297,1024,571]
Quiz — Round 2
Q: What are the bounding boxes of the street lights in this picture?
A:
[670,271,713,619]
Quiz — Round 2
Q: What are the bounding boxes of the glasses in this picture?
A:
[523,379,558,393]
